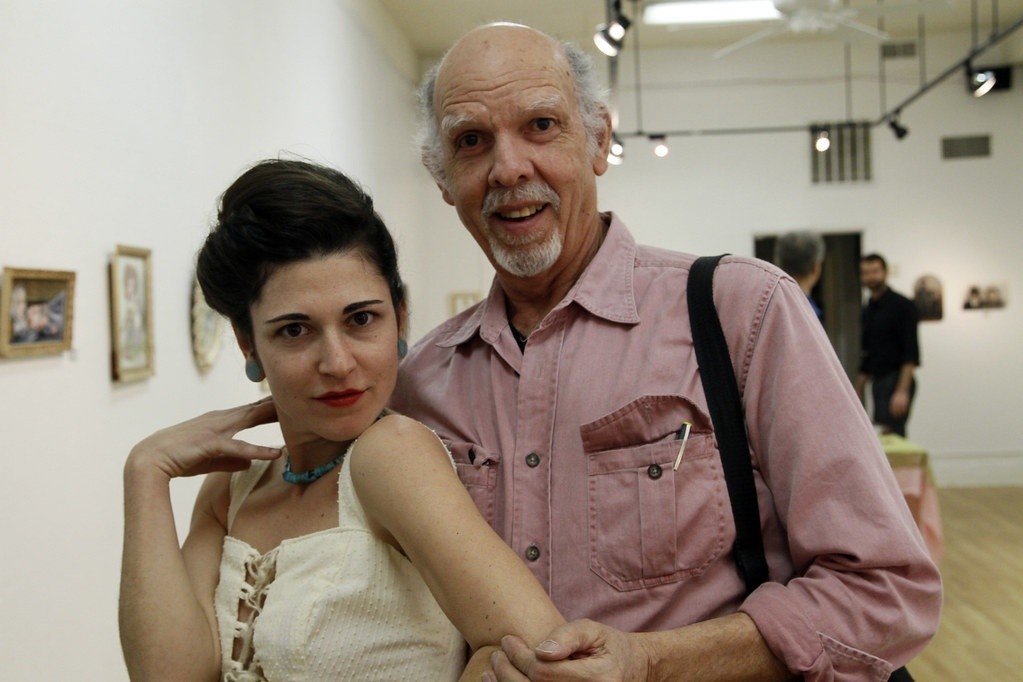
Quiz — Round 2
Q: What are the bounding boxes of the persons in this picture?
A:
[386,23,940,681]
[120,160,568,681]
[774,229,826,321]
[853,254,920,439]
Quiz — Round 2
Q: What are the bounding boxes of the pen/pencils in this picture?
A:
[674,421,695,474]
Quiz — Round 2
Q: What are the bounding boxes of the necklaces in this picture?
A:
[281,409,387,484]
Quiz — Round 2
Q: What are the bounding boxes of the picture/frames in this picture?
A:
[190,275,226,374]
[109,243,157,383]
[0,266,77,360]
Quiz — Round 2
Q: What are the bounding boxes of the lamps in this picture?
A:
[966,70,998,98]
[591,15,630,59]
